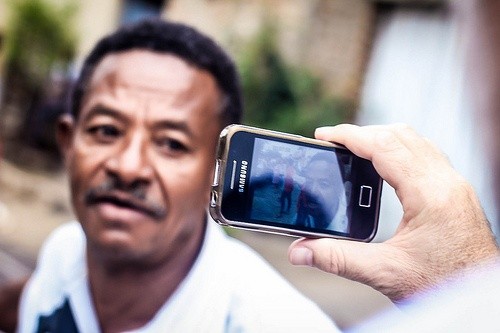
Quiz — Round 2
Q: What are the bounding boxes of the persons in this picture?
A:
[285,0,499,333]
[1,19,341,333]
[255,144,353,235]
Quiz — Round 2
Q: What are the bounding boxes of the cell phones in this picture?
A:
[208,123,383,246]
